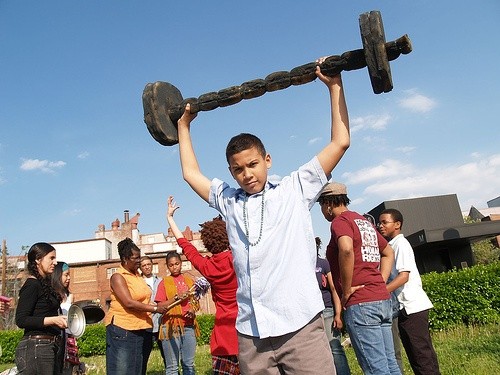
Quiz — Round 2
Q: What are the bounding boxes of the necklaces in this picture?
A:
[243,189,265,246]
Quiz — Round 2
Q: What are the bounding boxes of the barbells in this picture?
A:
[142,10,414,146]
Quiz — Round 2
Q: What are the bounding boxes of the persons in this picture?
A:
[177,56,351,375]
[105,237,168,375]
[48,261,86,375]
[154,250,200,375]
[140,256,181,375]
[379,208,441,375]
[316,182,402,375]
[315,237,352,375]
[15,242,68,375]
[167,195,240,375]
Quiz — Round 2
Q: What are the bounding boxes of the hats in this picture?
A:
[320,182,347,197]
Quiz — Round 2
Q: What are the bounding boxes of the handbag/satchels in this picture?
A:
[64,334,80,365]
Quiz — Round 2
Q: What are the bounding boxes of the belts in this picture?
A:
[20,334,62,342]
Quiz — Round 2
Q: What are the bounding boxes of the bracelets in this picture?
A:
[153,306,157,314]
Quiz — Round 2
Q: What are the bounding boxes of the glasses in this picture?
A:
[377,221,396,227]
